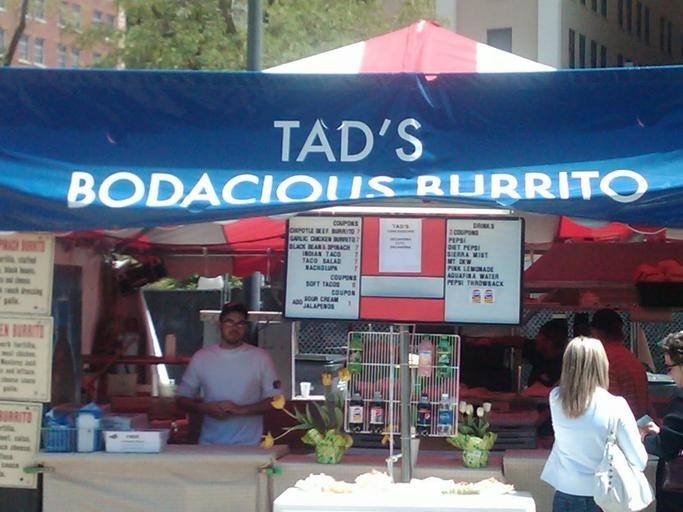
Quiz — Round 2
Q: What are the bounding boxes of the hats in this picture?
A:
[223,301,245,310]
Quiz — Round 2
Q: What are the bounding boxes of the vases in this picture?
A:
[299,429,353,463]
[449,435,498,468]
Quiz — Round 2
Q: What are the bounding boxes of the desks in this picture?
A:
[42,450,659,512]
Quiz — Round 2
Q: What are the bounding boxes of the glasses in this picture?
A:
[664,363,680,373]
[222,320,248,328]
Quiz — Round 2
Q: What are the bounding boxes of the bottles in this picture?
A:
[436,335,451,379]
[417,334,434,378]
[368,391,385,432]
[435,393,452,436]
[348,391,364,432]
[416,394,431,436]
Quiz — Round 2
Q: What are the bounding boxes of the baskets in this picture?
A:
[39,428,75,452]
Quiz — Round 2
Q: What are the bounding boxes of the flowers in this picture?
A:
[257,367,355,452]
[456,397,495,440]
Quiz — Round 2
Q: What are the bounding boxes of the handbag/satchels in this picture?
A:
[594,434,655,512]
[660,456,682,494]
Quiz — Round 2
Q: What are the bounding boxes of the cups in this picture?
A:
[299,382,311,398]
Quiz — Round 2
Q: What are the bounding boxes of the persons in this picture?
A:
[173,299,283,445]
[637,330,683,511]
[584,307,657,421]
[538,336,648,511]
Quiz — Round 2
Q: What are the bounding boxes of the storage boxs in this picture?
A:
[100,426,172,456]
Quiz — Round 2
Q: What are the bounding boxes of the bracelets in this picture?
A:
[640,430,649,438]
